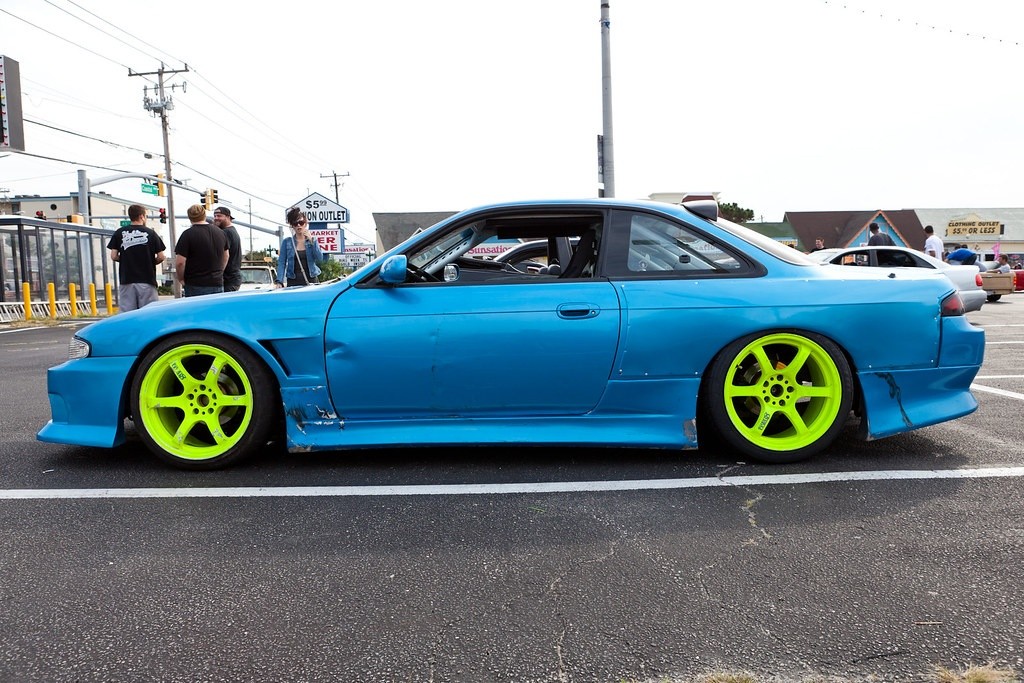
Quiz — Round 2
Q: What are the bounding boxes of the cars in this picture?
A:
[808,246,987,316]
[34,198,987,472]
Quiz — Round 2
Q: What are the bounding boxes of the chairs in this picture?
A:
[558,229,597,279]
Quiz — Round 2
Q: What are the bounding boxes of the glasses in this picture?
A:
[292,221,306,227]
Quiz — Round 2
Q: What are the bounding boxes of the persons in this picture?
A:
[925,226,946,261]
[788,237,828,255]
[944,245,986,272]
[868,223,896,246]
[986,253,1023,274]
[107,205,166,314]
[275,210,323,289]
[174,205,242,297]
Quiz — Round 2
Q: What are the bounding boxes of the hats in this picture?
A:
[187,204,206,222]
[214,207,235,219]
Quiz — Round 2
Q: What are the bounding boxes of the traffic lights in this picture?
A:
[200,193,206,209]
[159,209,166,223]
[214,190,218,203]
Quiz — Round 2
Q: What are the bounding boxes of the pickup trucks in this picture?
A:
[980,269,1017,302]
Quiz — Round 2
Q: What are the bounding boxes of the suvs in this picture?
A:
[238,257,278,293]
